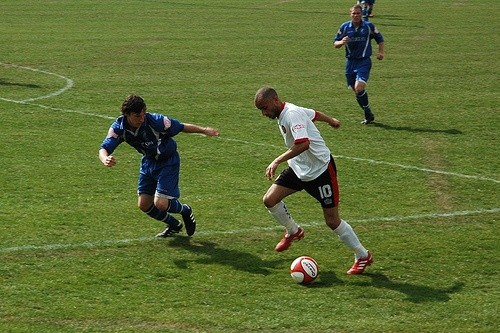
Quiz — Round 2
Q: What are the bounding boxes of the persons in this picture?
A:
[254,86,374,274]
[98,93,219,236]
[333,3,385,124]
[356,0,375,21]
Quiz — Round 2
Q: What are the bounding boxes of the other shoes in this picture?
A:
[361,115,375,124]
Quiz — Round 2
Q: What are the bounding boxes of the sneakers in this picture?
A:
[346,250,374,274]
[156,220,183,237]
[183,203,196,236]
[274,226,305,251]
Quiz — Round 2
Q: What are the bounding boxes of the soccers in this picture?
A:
[290,256,319,284]
